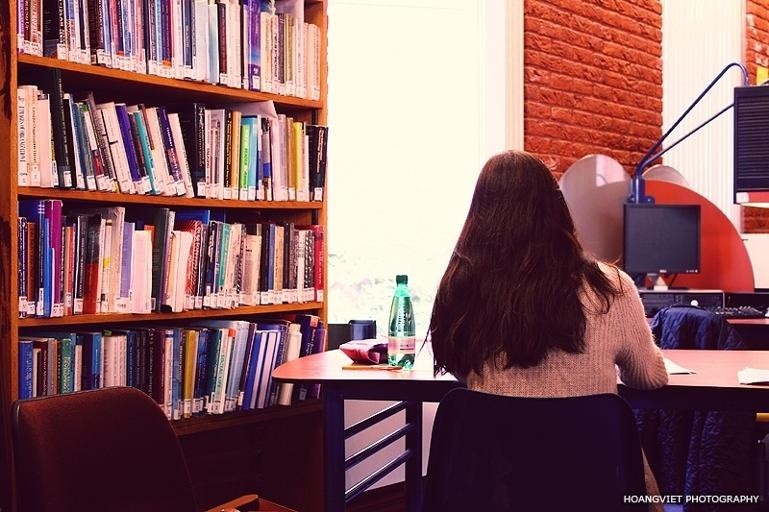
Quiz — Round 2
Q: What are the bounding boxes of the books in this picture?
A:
[16,0,329,420]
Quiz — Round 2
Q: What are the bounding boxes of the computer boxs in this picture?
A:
[639,289,725,318]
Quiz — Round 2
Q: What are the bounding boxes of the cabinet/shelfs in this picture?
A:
[0,1,331,510]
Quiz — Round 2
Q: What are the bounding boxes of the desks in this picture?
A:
[275,337,769,510]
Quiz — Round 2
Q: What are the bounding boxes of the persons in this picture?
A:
[420,150,668,512]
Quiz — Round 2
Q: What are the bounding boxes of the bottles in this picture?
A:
[387,275,416,367]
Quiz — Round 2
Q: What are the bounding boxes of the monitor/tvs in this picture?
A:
[622,204,702,290]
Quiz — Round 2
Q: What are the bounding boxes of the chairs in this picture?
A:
[9,390,295,511]
[420,387,649,509]
